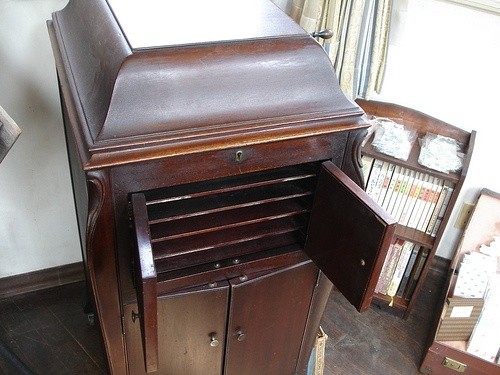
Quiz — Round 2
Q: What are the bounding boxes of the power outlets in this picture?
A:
[454,201,474,231]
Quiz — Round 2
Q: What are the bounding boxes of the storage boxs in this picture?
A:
[418,187,500,375]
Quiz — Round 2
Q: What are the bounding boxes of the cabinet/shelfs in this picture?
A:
[46,0,372,375]
[354,97,478,325]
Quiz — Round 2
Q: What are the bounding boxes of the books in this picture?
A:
[306,326,329,375]
[359,155,456,301]
